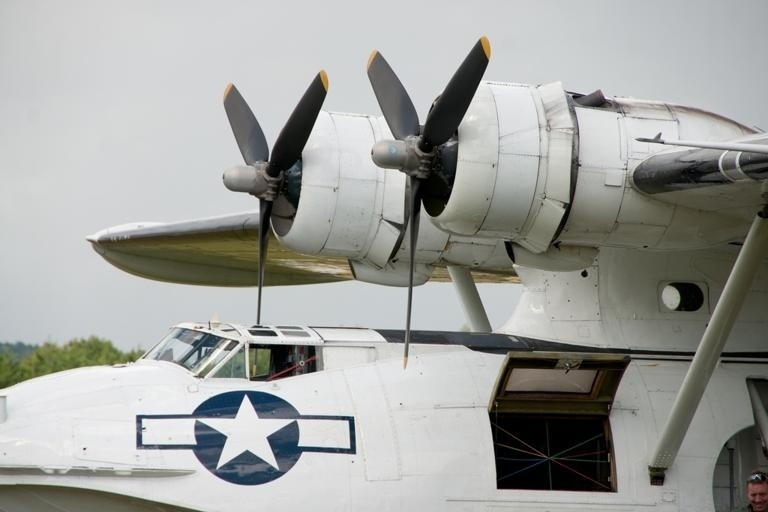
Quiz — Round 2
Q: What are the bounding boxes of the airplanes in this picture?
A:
[1,35,768,511]
